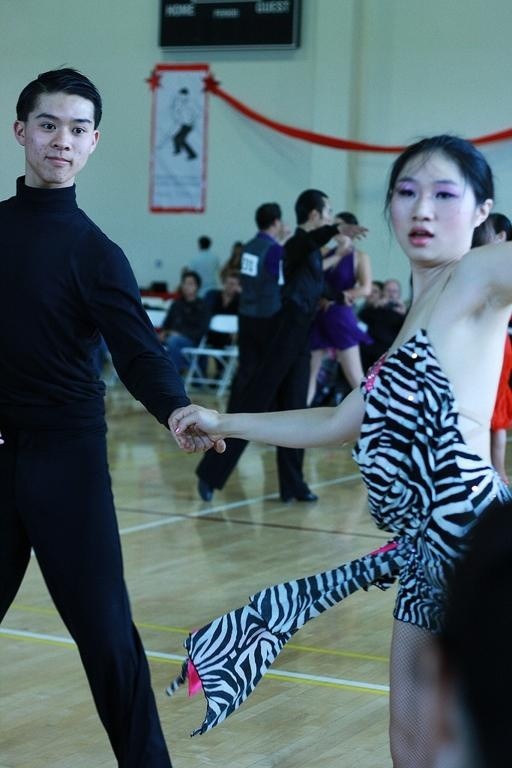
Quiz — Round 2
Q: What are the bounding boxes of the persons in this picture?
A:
[281,213,369,405]
[477,213,511,488]
[410,496,511,768]
[176,133,511,766]
[195,190,367,502]
[0,59,229,767]
[233,201,290,357]
[158,237,411,405]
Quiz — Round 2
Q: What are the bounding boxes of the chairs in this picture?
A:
[181,312,243,401]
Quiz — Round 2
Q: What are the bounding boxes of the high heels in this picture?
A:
[282,491,319,505]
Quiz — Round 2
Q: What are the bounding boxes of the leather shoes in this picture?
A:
[199,477,213,501]
